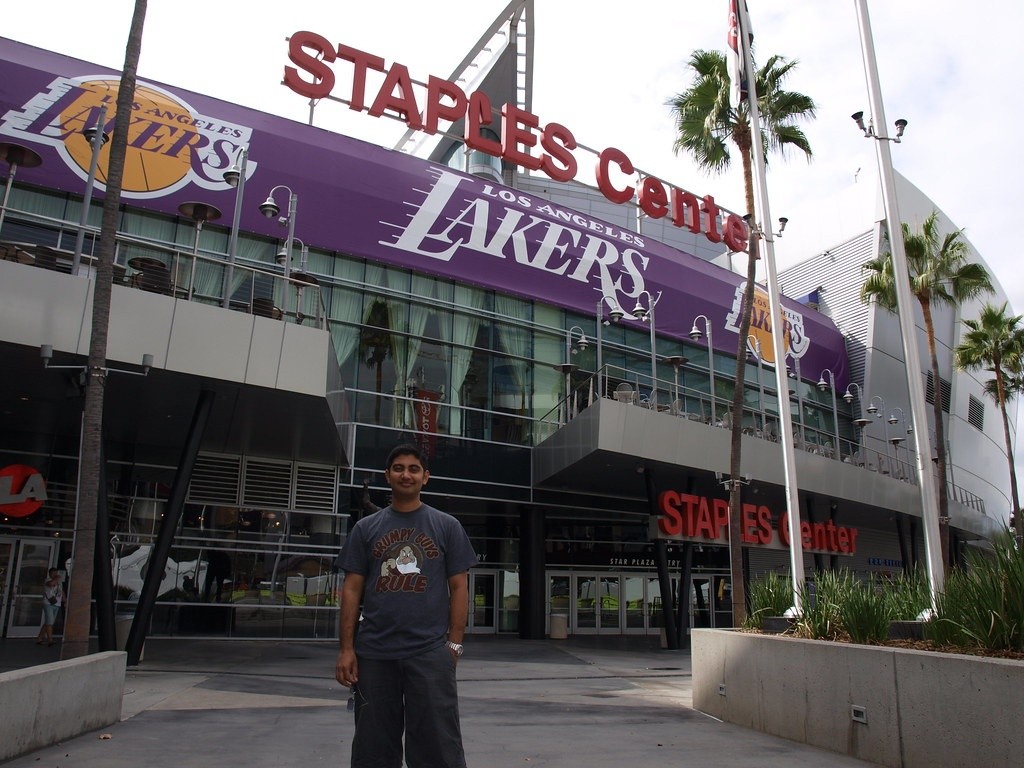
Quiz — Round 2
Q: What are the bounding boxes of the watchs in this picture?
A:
[445,640,464,656]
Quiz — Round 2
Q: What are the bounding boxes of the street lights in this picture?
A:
[843,383,867,468]
[565,325,589,423]
[222,148,248,308]
[785,352,806,450]
[868,395,891,477]
[632,290,658,411]
[888,407,911,482]
[745,335,766,440]
[690,315,716,426]
[597,296,625,397]
[260,186,298,320]
[817,369,841,462]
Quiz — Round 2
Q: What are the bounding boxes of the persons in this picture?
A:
[335,444,479,768]
[36,567,67,648]
[204,521,232,602]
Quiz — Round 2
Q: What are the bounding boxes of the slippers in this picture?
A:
[37,641,47,645]
[48,641,57,648]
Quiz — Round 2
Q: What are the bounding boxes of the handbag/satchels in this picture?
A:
[48,596,58,606]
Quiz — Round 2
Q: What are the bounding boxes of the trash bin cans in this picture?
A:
[550,611,568,639]
[115,610,145,662]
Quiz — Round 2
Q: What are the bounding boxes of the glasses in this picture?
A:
[347,682,368,711]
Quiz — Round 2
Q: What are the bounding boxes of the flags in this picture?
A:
[726,0,754,108]
